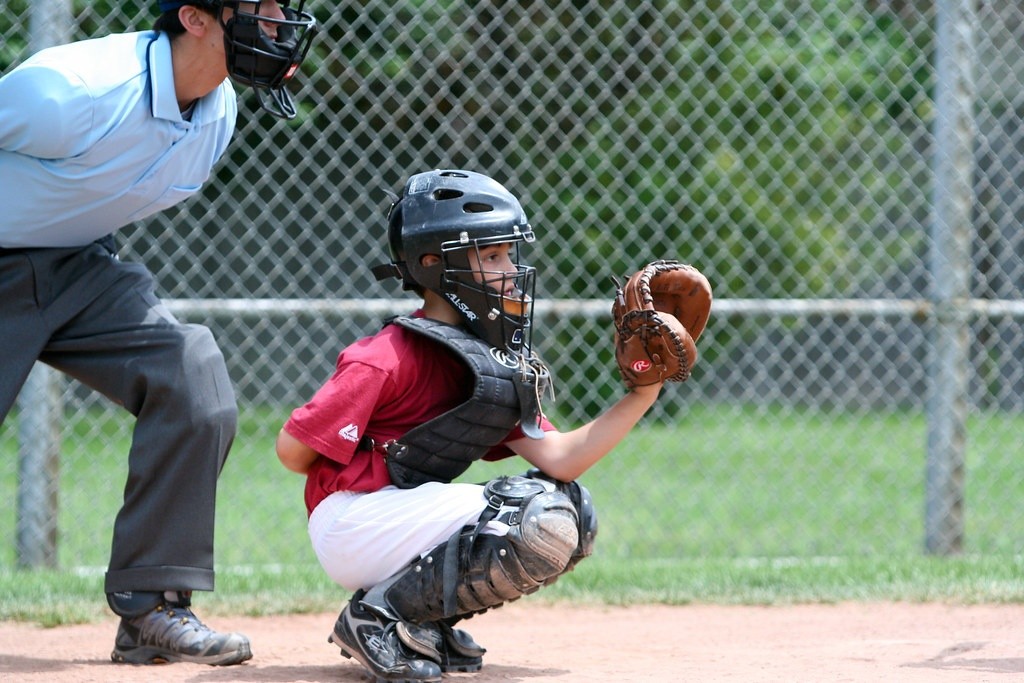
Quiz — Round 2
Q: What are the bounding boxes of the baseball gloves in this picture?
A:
[605,257,716,389]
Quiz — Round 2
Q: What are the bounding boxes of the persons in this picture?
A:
[0,0,319,665]
[277,169,711,683]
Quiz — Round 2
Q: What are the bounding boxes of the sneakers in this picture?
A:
[327,588,487,683]
[106,589,253,666]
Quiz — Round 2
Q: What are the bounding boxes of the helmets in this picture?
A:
[159,0,317,120]
[370,168,537,358]
